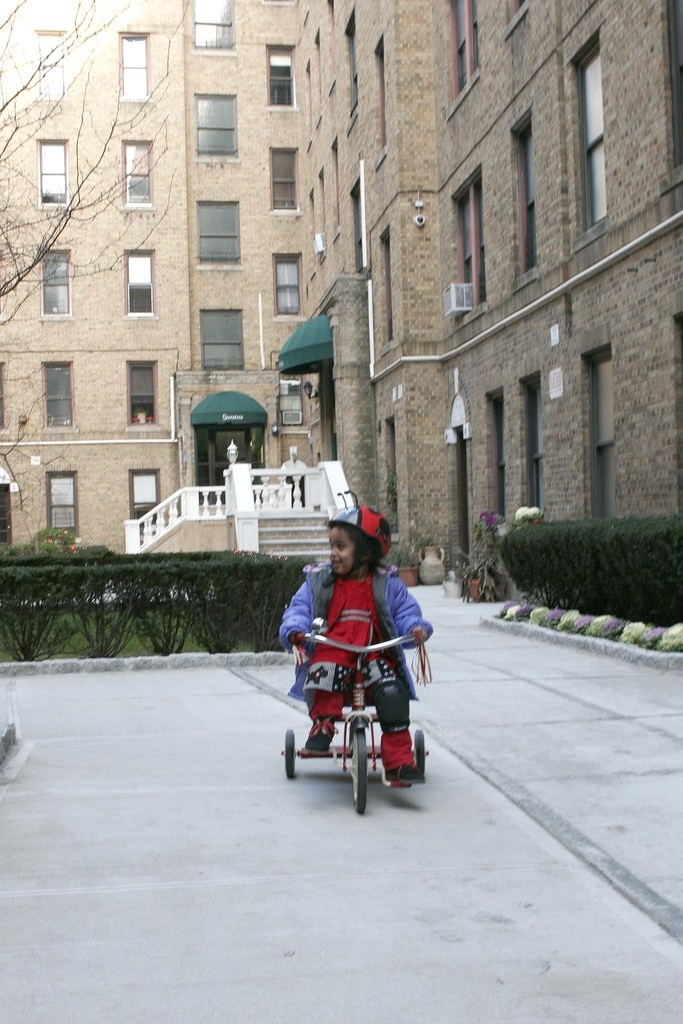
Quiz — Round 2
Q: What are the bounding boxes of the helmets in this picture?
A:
[327,491,392,557]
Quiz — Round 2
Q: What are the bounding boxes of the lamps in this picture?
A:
[226,438,238,463]
[303,380,317,400]
[272,423,279,435]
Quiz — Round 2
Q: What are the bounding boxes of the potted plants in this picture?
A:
[386,525,432,587]
[134,408,148,424]
[460,556,506,603]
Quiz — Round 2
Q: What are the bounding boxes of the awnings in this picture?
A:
[189,392,267,424]
[279,316,334,374]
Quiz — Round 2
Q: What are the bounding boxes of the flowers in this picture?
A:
[479,511,503,527]
[514,504,542,524]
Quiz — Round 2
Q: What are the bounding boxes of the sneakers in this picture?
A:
[304,716,337,755]
[384,763,427,785]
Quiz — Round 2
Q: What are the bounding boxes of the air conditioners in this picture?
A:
[312,232,324,254]
[282,411,302,424]
[442,282,473,318]
[280,381,299,395]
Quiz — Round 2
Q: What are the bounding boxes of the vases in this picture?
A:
[528,518,540,525]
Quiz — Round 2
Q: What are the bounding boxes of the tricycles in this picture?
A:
[281,617,430,814]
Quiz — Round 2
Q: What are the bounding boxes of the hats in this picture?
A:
[290,446,297,453]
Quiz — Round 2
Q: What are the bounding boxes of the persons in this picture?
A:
[280,506,434,784]
